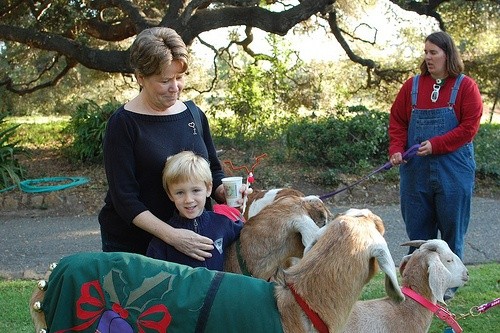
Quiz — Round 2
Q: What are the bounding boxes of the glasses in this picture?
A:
[430,83,441,103]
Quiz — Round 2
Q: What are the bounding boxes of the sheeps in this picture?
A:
[30,208,405,333]
[344,238,470,333]
[224,186,334,283]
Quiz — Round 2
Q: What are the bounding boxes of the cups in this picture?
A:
[221,177,244,207]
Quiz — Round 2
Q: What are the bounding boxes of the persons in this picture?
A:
[144,151,245,272]
[388,31,484,300]
[98,25,254,261]
